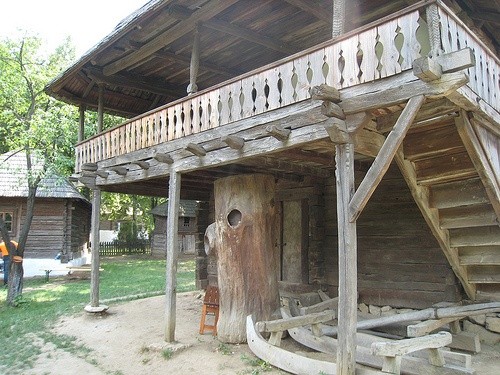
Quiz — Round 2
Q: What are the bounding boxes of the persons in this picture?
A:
[0,237,19,288]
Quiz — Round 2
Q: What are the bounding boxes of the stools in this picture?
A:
[198,304,220,336]
